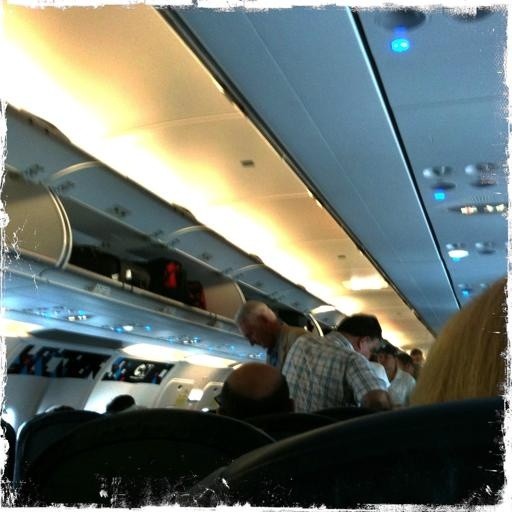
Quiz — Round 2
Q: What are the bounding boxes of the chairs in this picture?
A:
[2,397,502,506]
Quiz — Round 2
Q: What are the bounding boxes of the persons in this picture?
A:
[45,405,77,413]
[107,395,135,414]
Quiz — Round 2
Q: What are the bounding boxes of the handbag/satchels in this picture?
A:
[69,243,207,311]
